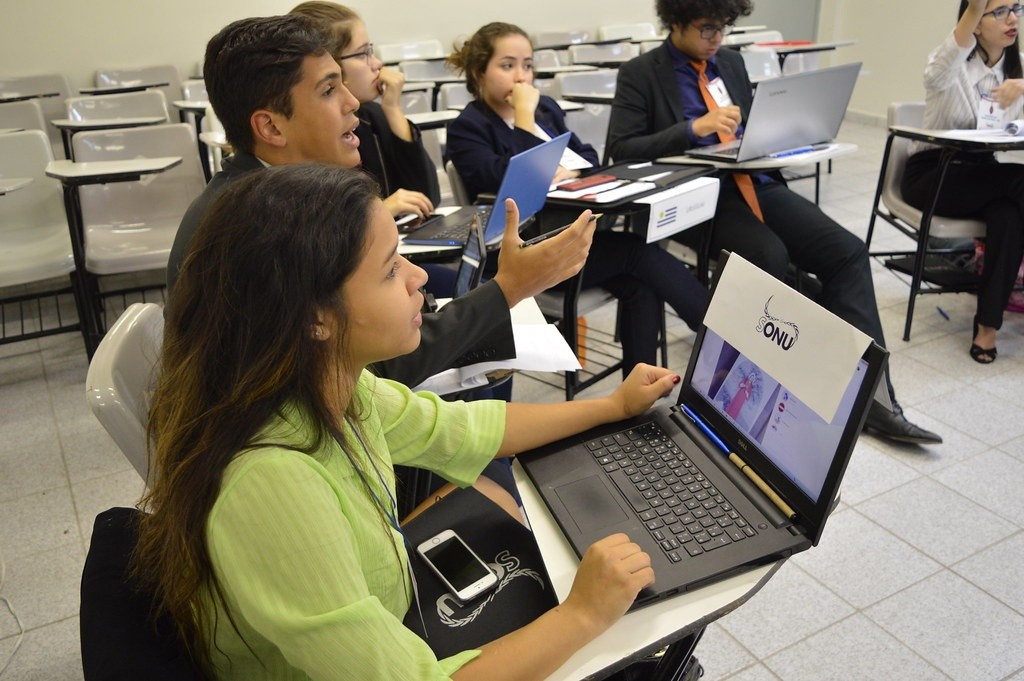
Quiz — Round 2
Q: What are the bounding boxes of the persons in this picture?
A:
[447,21,713,374]
[901,1,1024,363]
[166,15,597,517]
[290,1,459,296]
[606,0,944,445]
[131,159,680,681]
[726,373,755,420]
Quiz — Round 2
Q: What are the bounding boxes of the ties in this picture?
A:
[690,59,765,223]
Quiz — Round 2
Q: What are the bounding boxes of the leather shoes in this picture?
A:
[862,393,942,444]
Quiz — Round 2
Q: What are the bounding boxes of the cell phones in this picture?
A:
[397,214,444,234]
[557,173,617,193]
[417,529,500,605]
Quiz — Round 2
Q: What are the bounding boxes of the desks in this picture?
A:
[532,63,595,77]
[377,58,400,67]
[397,203,538,259]
[627,34,663,48]
[592,52,630,70]
[412,51,451,68]
[532,158,712,218]
[52,114,160,154]
[733,22,769,34]
[505,424,843,667]
[557,99,584,130]
[437,73,469,84]
[388,79,435,98]
[889,118,1021,156]
[46,154,180,274]
[652,140,860,173]
[0,175,33,213]
[542,41,587,53]
[562,87,620,105]
[404,107,458,154]
[761,36,855,73]
[0,88,56,105]
[198,128,233,176]
[77,79,168,103]
[591,35,627,51]
[175,95,213,133]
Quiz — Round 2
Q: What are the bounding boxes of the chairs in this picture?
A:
[0,21,1023,681]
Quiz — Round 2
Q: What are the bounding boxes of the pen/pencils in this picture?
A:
[679,403,730,458]
[728,452,796,522]
[519,212,608,249]
[936,305,950,321]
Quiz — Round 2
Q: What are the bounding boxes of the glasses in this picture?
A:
[687,18,735,39]
[338,41,376,65]
[983,3,1024,20]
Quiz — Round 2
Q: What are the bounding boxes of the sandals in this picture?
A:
[969,314,998,362]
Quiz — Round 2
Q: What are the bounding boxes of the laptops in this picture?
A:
[452,209,487,301]
[403,130,573,247]
[684,61,863,163]
[515,249,889,614]
